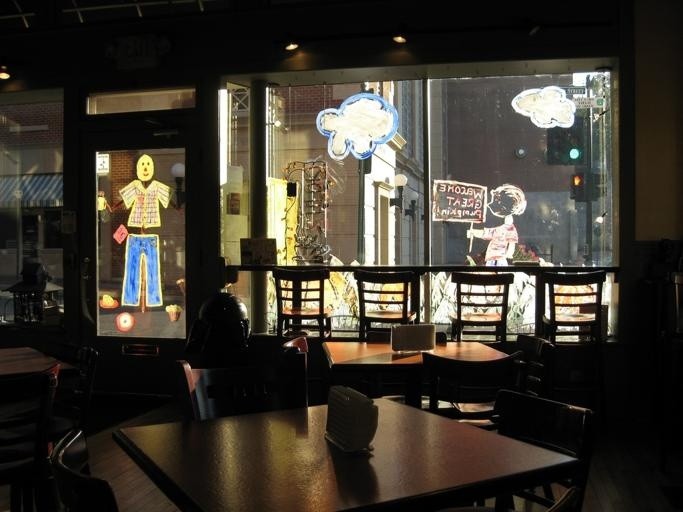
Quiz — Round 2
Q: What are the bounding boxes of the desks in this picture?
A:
[0,346,61,402]
[113,396,578,511]
[323,340,513,419]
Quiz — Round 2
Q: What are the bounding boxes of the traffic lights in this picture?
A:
[566,116,583,165]
[548,127,565,165]
[570,173,583,201]
[593,173,601,202]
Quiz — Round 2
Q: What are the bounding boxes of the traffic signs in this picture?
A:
[571,95,605,109]
[554,85,585,95]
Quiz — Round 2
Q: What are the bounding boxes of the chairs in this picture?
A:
[422,349,528,432]
[279,334,312,412]
[46,427,118,512]
[0,363,61,512]
[512,332,557,395]
[532,270,606,361]
[468,389,595,512]
[271,269,335,354]
[181,358,296,419]
[450,271,516,350]
[352,270,419,343]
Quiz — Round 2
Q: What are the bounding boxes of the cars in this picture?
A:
[465,243,539,266]
